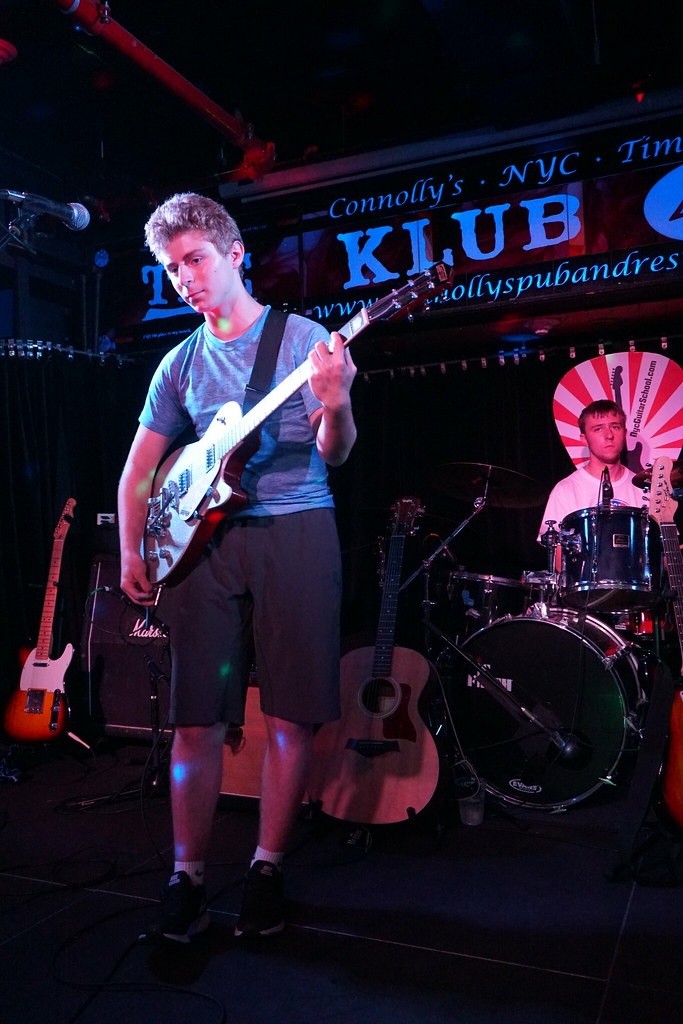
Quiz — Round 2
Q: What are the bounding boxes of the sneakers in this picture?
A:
[137,871,207,948]
[234,860,286,939]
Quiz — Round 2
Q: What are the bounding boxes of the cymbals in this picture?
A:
[432,461,552,510]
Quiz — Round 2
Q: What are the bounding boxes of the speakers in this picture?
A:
[82,555,174,739]
[213,671,313,806]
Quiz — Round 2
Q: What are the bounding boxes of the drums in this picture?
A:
[559,507,661,612]
[522,574,564,610]
[445,572,527,643]
[447,608,643,809]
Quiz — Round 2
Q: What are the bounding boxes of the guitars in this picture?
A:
[144,259,452,588]
[3,498,77,744]
[309,495,442,826]
[641,456,683,842]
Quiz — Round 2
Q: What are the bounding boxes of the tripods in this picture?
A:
[64,654,171,812]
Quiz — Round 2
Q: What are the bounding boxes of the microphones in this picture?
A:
[602,467,614,500]
[0,189,90,231]
[102,584,116,593]
[440,538,456,562]
[555,733,581,760]
[224,727,243,745]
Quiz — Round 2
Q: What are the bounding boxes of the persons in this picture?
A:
[536,400,651,585]
[117,191,357,943]
